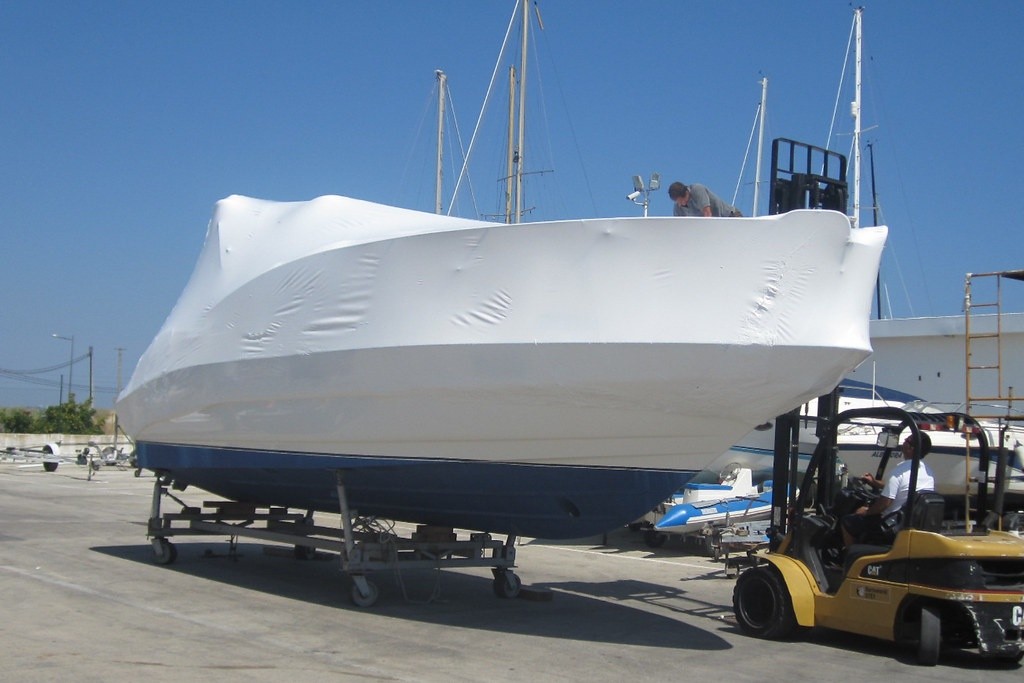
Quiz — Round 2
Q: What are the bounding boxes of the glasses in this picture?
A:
[904,438,912,446]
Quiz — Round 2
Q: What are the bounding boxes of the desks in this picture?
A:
[722,535,769,575]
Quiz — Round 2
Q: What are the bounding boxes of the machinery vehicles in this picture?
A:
[732,137,1024,669]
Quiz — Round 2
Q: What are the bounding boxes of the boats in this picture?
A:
[113,193,891,540]
[694,376,1024,511]
[652,461,803,534]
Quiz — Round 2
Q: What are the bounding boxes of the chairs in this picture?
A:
[848,490,945,563]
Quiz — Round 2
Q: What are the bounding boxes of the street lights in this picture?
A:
[52,334,74,403]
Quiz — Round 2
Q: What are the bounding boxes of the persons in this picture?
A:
[669,181,743,217]
[831,431,934,568]
